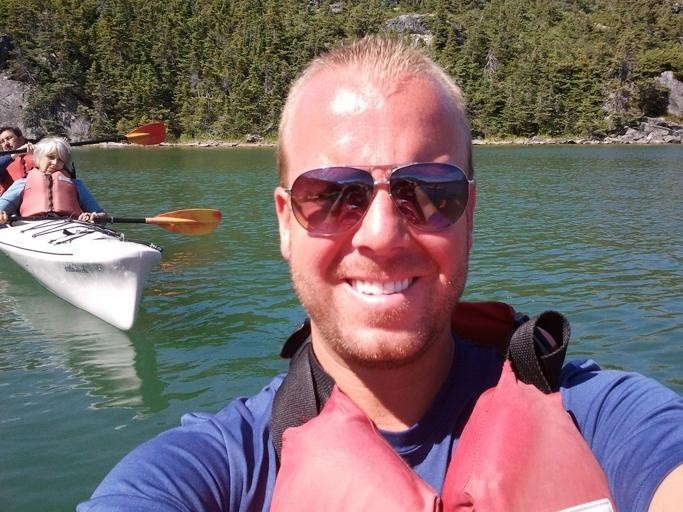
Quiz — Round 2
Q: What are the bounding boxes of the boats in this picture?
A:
[0,258,163,417]
[0,211,163,332]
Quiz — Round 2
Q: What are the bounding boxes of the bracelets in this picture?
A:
[92,211,101,222]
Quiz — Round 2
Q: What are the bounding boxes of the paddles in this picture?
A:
[9,208,223,236]
[0,122,167,155]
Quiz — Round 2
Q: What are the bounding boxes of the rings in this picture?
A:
[83,212,90,217]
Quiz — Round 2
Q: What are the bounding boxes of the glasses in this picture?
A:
[283,160,478,236]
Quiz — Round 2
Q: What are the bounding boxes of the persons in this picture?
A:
[0,126,48,201]
[0,136,111,228]
[73,34,682,512]
